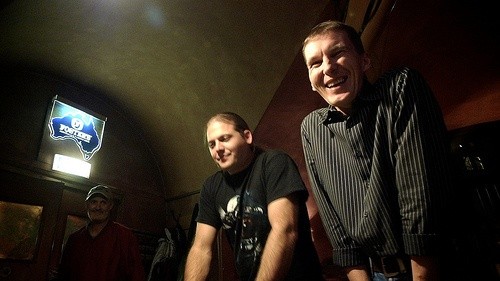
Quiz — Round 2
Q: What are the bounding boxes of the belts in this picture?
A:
[366,255,412,277]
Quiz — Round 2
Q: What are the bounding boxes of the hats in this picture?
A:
[85,184,113,201]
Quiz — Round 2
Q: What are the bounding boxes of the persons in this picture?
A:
[301,21,463,281]
[60,184,144,281]
[184,112,324,281]
